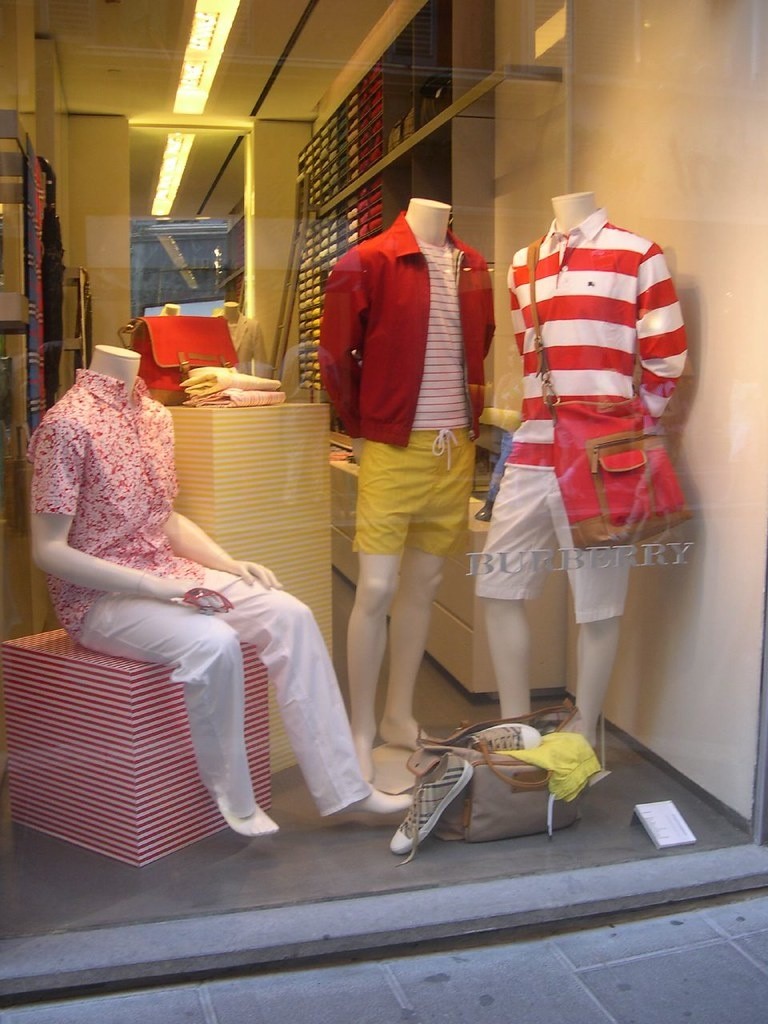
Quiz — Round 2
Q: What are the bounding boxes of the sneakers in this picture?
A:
[448,721,543,752]
[388,751,474,867]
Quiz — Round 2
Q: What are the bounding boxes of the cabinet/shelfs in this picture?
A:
[329,459,568,703]
[299,0,573,493]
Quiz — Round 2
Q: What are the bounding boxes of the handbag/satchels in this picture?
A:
[116,315,240,405]
[406,697,578,843]
[541,380,695,552]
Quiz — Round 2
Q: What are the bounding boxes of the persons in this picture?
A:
[211,302,268,377]
[25,345,413,837]
[318,197,494,784]
[475,190,687,749]
